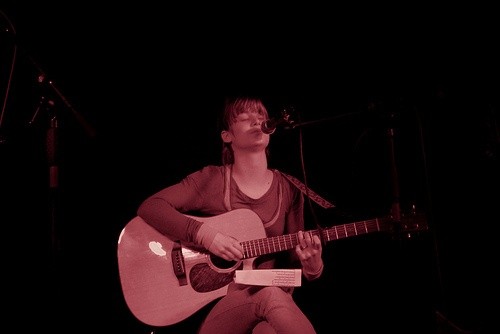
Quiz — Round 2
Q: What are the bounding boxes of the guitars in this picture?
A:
[117,202,428,327]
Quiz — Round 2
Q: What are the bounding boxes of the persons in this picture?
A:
[137,91,326,334]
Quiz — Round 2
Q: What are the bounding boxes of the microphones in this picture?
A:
[261,110,290,134]
